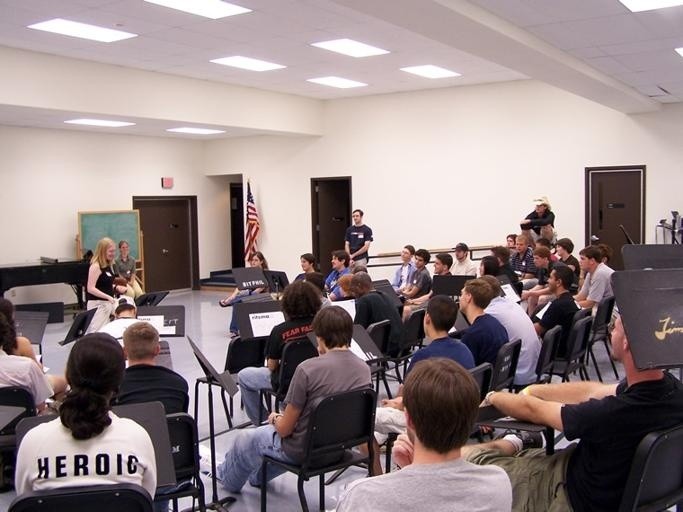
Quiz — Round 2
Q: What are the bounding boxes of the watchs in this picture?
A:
[270,414,283,425]
[485,390,495,404]
[457,315,683,511]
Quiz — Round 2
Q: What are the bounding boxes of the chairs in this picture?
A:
[491,338,522,392]
[155,413,206,511]
[388,309,425,383]
[535,325,561,383]
[569,309,591,380]
[8,485,153,512]
[0,387,34,448]
[262,385,376,512]
[552,316,590,382]
[367,320,394,399]
[466,363,492,404]
[618,425,683,512]
[588,296,619,382]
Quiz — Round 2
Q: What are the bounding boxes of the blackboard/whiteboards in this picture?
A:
[80,210,141,263]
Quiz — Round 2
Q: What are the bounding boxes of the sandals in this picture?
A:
[219,299,233,307]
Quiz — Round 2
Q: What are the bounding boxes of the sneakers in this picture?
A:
[199,454,220,485]
[502,431,547,452]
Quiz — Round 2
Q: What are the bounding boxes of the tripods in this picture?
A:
[180,384,236,512]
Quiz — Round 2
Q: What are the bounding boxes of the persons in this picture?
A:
[98,296,146,370]
[506,234,518,256]
[219,252,270,338]
[521,246,579,316]
[519,195,558,246]
[490,246,522,298]
[353,266,369,274]
[303,272,331,311]
[480,276,541,393]
[391,244,415,294]
[87,237,137,319]
[16,332,156,503]
[595,243,613,267]
[556,238,580,292]
[401,253,452,322]
[237,283,323,426]
[357,295,476,477]
[457,279,510,366]
[511,234,539,291]
[0,298,68,403]
[195,306,372,492]
[346,210,373,270]
[328,357,513,511]
[396,249,431,303]
[479,256,520,304]
[350,271,413,358]
[114,240,143,300]
[535,239,558,262]
[294,254,320,282]
[112,322,189,511]
[574,246,616,319]
[450,243,477,276]
[334,274,357,301]
[0,313,55,411]
[324,250,353,300]
[533,263,582,336]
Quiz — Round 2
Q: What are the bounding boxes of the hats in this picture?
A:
[532,198,551,208]
[452,242,470,250]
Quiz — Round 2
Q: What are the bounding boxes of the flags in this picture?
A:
[245,181,259,263]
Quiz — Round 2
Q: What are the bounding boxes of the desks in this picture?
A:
[1,259,88,314]
